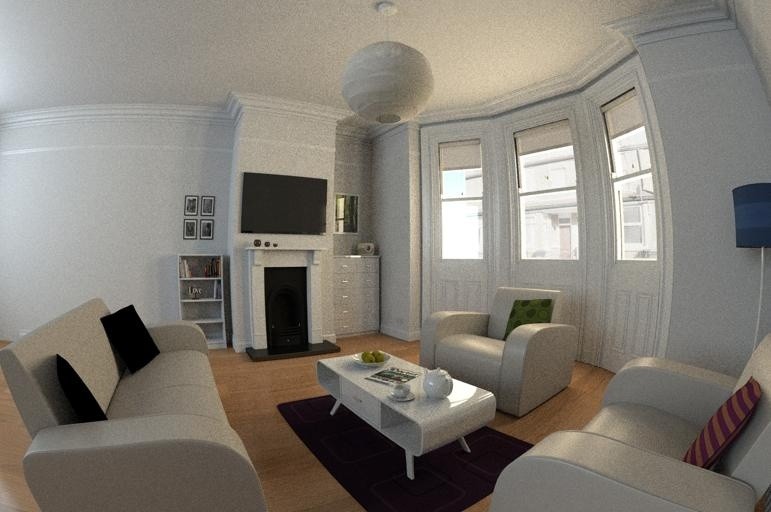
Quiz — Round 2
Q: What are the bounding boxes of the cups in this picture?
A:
[392,384,410,398]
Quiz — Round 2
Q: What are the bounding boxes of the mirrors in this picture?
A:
[336,195,345,220]
[335,194,358,234]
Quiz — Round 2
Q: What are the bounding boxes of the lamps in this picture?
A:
[340,1,434,124]
[732,183,771,350]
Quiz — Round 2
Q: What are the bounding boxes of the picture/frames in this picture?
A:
[183,219,197,240]
[201,196,215,216]
[184,195,198,216]
[200,219,214,240]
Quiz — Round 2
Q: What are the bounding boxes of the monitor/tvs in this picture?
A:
[240,172,328,236]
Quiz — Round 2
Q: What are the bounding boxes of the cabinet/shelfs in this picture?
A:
[334,255,379,338]
[177,254,227,348]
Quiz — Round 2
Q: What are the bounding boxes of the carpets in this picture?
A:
[277,394,535,512]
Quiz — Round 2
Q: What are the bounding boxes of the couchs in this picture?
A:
[490,334,771,512]
[418,286,577,417]
[0,299,267,512]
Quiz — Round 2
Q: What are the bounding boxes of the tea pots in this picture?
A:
[423,366,453,401]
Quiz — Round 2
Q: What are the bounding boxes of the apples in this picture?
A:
[361,350,386,362]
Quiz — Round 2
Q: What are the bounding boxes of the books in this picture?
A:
[179,257,192,278]
[364,366,423,386]
[214,280,222,299]
[205,257,220,277]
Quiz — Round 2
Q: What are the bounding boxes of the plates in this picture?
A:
[351,351,391,368]
[388,391,415,402]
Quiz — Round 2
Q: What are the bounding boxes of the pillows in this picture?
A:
[504,299,552,340]
[100,304,159,375]
[55,353,108,423]
[683,376,762,468]
